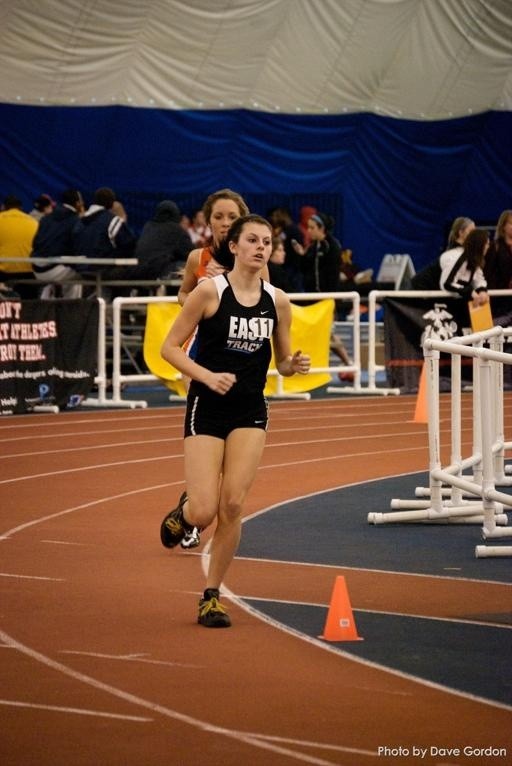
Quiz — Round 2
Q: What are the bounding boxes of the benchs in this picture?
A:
[0,273,183,379]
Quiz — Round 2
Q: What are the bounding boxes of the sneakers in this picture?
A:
[161,492,185,549]
[181,527,200,549]
[196,587,233,629]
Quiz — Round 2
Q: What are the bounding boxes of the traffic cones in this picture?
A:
[319,574,364,644]
[412,362,432,424]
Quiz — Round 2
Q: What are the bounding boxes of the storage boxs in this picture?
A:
[358,339,387,371]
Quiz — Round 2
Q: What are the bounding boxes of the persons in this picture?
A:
[177,188,272,549]
[440,210,512,392]
[160,213,311,629]
